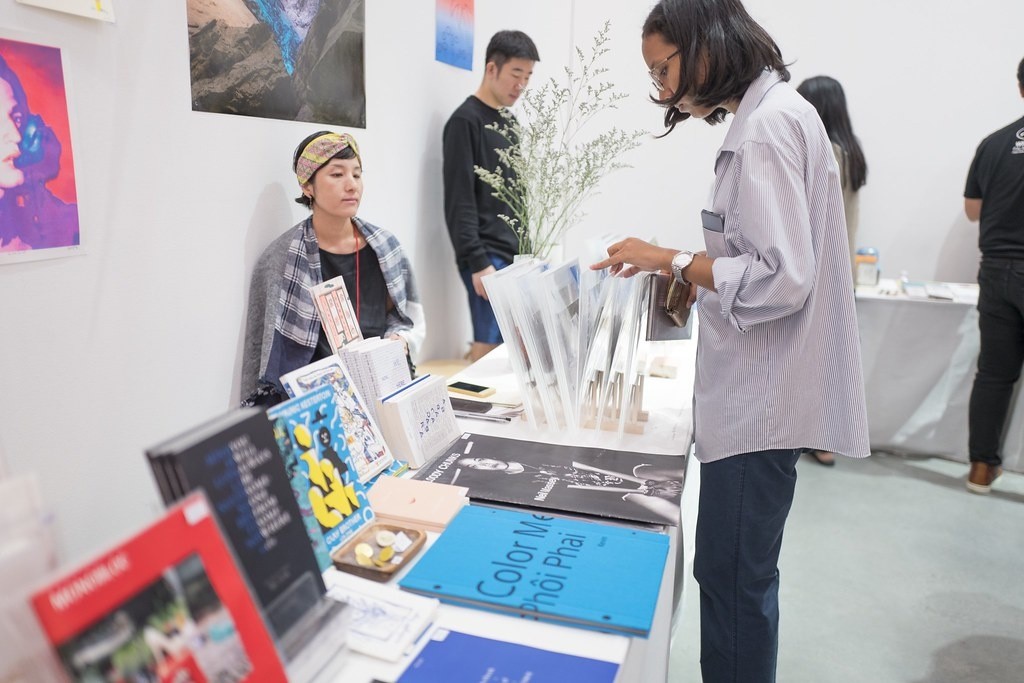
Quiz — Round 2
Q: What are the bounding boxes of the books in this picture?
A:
[0,252,695,683]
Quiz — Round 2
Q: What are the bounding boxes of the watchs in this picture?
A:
[671,250,697,285]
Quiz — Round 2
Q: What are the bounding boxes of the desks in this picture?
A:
[308,281,1024,683]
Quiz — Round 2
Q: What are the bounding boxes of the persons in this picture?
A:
[454,454,684,525]
[590,1,871,683]
[442,30,540,342]
[239,132,426,407]
[795,75,867,465]
[963,57,1024,491]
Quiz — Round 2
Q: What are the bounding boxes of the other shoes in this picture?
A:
[812,450,834,465]
[966,461,1004,492]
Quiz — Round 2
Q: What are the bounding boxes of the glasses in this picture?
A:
[648,49,681,92]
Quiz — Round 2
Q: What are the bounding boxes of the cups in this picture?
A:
[854,248,881,285]
